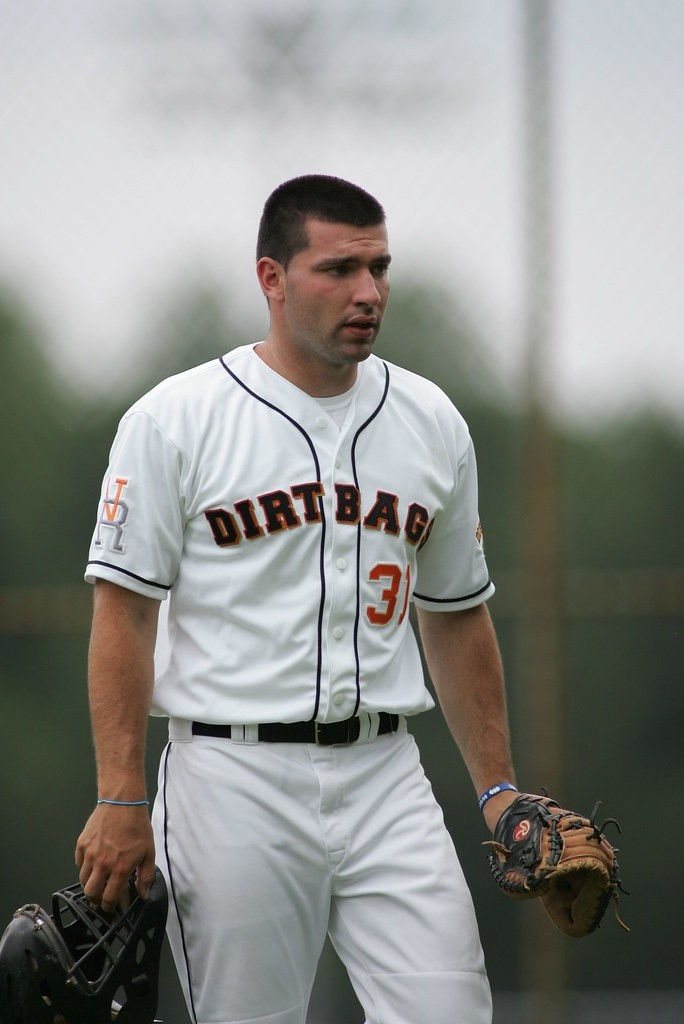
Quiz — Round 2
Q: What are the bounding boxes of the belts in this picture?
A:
[193,713,400,748]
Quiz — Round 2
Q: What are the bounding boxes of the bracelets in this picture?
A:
[96,798,150,806]
[478,782,518,812]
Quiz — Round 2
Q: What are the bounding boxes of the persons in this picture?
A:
[75,174,523,1024]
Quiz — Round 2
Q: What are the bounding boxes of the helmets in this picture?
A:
[0,864,169,1024]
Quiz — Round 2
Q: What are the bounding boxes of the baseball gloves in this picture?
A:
[484,790,622,943]
